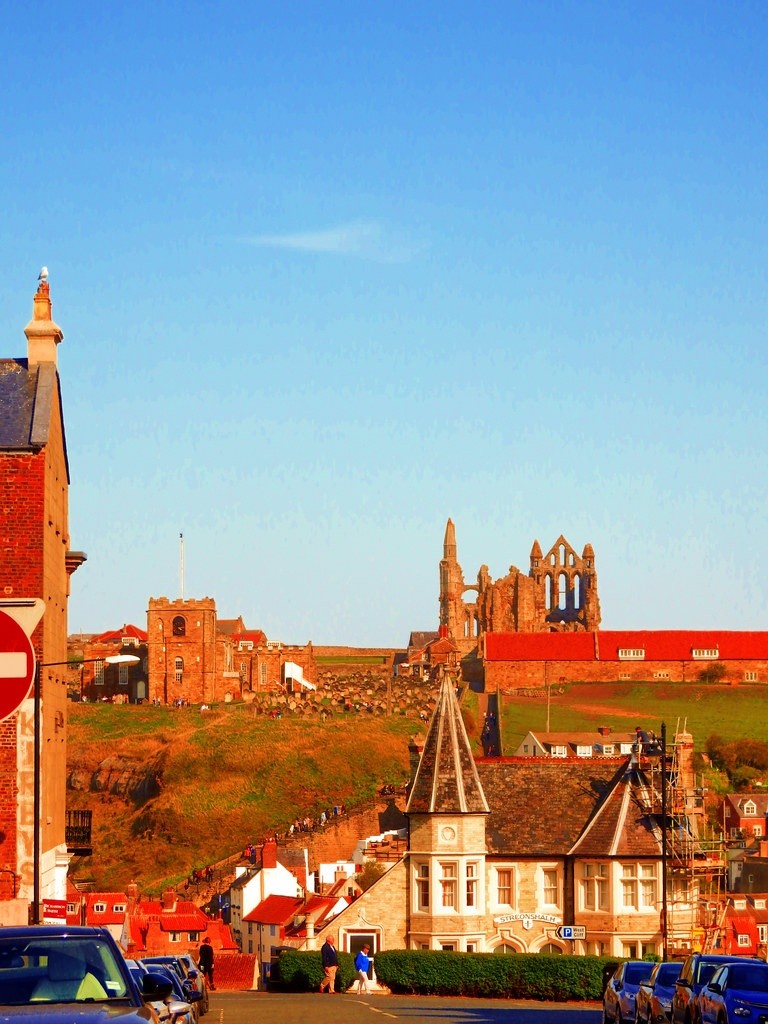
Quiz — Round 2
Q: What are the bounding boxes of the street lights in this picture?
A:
[642,722,668,961]
[32,654,143,924]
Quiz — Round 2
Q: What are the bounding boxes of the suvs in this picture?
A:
[0,922,173,1024]
[125,955,210,1024]
[671,951,764,1024]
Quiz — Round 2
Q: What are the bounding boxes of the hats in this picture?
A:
[363,943,370,950]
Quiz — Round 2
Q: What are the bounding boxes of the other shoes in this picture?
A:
[319,985,324,993]
[366,992,373,995]
[329,991,337,994]
[210,986,216,990]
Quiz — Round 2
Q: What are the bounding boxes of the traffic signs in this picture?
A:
[555,925,586,939]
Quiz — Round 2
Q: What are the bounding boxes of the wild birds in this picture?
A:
[37,266,48,281]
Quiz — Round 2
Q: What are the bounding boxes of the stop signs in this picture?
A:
[659,910,664,933]
[0,610,35,722]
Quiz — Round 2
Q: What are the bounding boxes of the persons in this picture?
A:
[481,710,497,756]
[382,784,394,797]
[355,944,374,995]
[192,865,213,885]
[356,704,359,712]
[319,935,339,994]
[636,727,648,753]
[333,803,338,819]
[272,708,281,719]
[82,692,128,705]
[296,816,310,824]
[320,809,330,826]
[405,782,408,793]
[274,831,278,843]
[342,802,346,814]
[420,709,427,719]
[348,703,350,711]
[249,844,252,851]
[153,696,190,709]
[198,937,216,990]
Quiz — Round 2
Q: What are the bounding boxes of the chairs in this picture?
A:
[733,971,751,990]
[29,946,94,1001]
[699,965,716,984]
[626,970,642,985]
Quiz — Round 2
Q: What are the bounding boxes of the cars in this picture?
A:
[634,962,683,1024]
[697,962,768,1024]
[602,960,657,1024]
